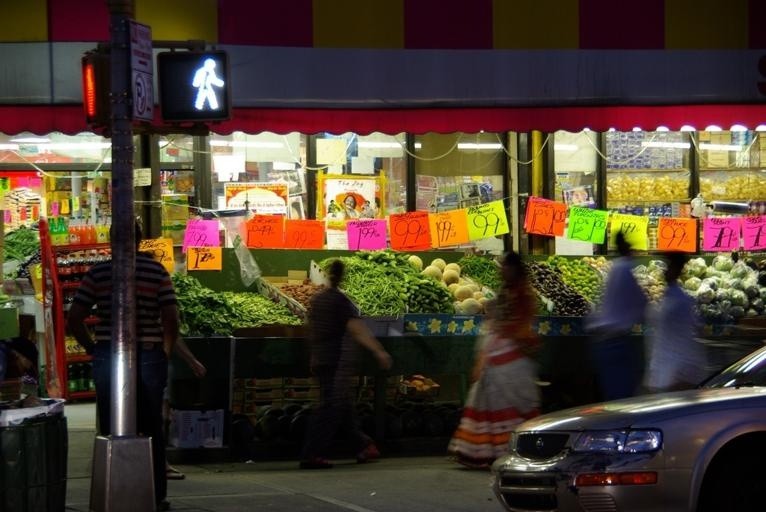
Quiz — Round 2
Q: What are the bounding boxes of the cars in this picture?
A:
[491,341,766,509]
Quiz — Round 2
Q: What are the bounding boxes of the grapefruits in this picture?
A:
[407,254,490,315]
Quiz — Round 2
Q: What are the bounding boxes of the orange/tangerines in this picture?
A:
[546,255,609,314]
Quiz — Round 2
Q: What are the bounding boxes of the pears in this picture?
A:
[646,278,668,304]
[583,254,607,271]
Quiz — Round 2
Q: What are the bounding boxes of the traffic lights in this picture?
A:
[166,55,228,118]
[81,57,105,118]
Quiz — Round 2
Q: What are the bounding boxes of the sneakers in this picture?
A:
[162,462,188,484]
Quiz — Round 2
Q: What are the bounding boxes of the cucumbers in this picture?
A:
[355,248,454,314]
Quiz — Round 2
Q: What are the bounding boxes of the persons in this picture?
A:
[641,262,713,393]
[361,200,374,218]
[584,240,648,401]
[448,251,542,471]
[302,260,393,469]
[328,200,341,212]
[66,221,180,512]
[340,195,363,220]
[137,251,207,481]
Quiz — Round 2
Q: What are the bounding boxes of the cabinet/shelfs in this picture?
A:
[38,220,112,403]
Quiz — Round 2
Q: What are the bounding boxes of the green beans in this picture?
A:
[317,255,402,320]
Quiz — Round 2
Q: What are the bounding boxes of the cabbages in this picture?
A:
[648,255,766,322]
